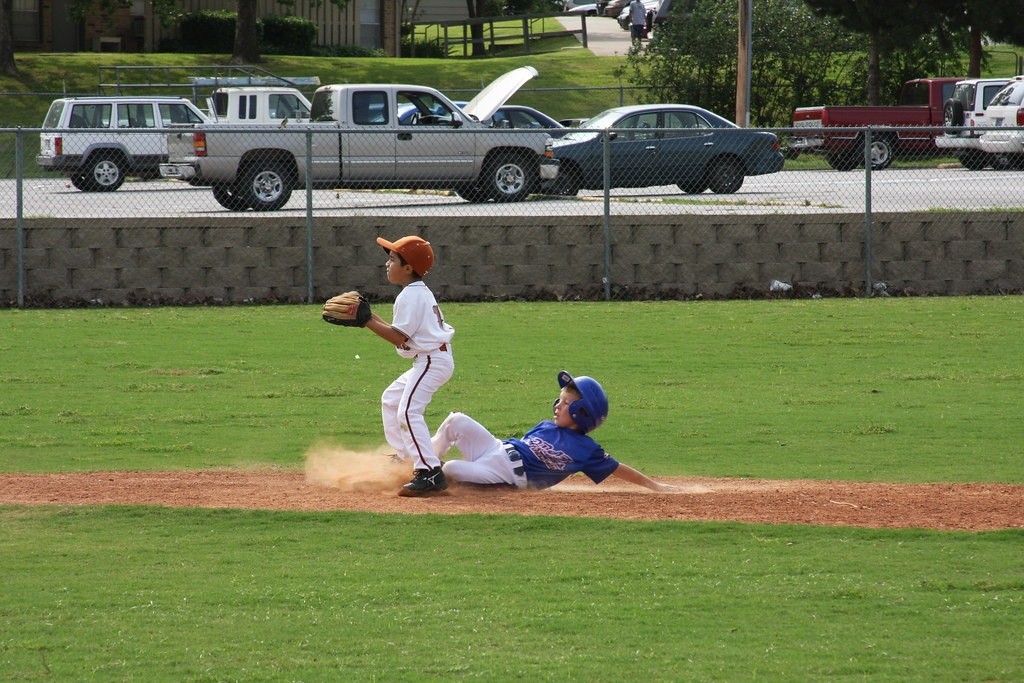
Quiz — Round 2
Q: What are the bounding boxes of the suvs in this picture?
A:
[35,95,216,191]
[935,76,1012,172]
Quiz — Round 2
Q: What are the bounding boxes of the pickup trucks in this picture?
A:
[101,65,555,210]
[791,77,973,172]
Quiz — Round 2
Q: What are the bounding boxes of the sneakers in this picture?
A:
[399,467,449,495]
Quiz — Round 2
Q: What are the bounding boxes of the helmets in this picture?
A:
[553,371,609,436]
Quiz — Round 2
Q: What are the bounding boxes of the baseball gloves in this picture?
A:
[319,288,373,329]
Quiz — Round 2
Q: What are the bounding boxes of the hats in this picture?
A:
[376,236,434,277]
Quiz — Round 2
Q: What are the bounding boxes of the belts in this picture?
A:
[414,344,448,359]
[502,441,524,476]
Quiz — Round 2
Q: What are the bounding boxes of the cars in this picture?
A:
[979,75,1023,170]
[567,0,654,29]
[368,99,592,130]
[545,104,785,194]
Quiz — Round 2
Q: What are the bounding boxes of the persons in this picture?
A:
[430,370,680,492]
[323,236,454,497]
[629,0,646,45]
